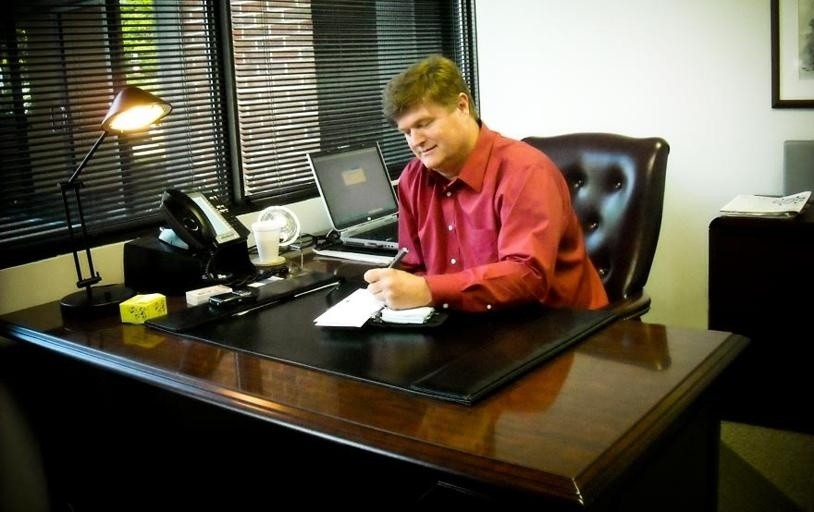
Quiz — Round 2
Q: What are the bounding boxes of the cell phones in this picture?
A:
[208,288,259,308]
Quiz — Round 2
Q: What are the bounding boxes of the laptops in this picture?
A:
[306,140,401,251]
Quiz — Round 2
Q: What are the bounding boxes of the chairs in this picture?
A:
[517,132,669,324]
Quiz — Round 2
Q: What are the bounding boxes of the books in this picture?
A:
[720,191,812,220]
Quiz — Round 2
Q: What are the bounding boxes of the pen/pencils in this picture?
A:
[386,247,409,268]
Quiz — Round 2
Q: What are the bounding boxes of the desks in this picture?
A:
[706,195,813,435]
[0,225,749,511]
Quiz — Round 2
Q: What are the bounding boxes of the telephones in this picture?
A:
[157,188,249,253]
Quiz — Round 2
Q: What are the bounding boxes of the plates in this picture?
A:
[251,256,286,267]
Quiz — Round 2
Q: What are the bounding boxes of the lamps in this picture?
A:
[56,85,171,324]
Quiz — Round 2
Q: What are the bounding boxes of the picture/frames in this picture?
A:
[768,0,814,110]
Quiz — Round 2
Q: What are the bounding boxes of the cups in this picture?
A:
[253,225,281,263]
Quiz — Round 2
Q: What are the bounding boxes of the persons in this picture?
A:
[364,54,609,313]
